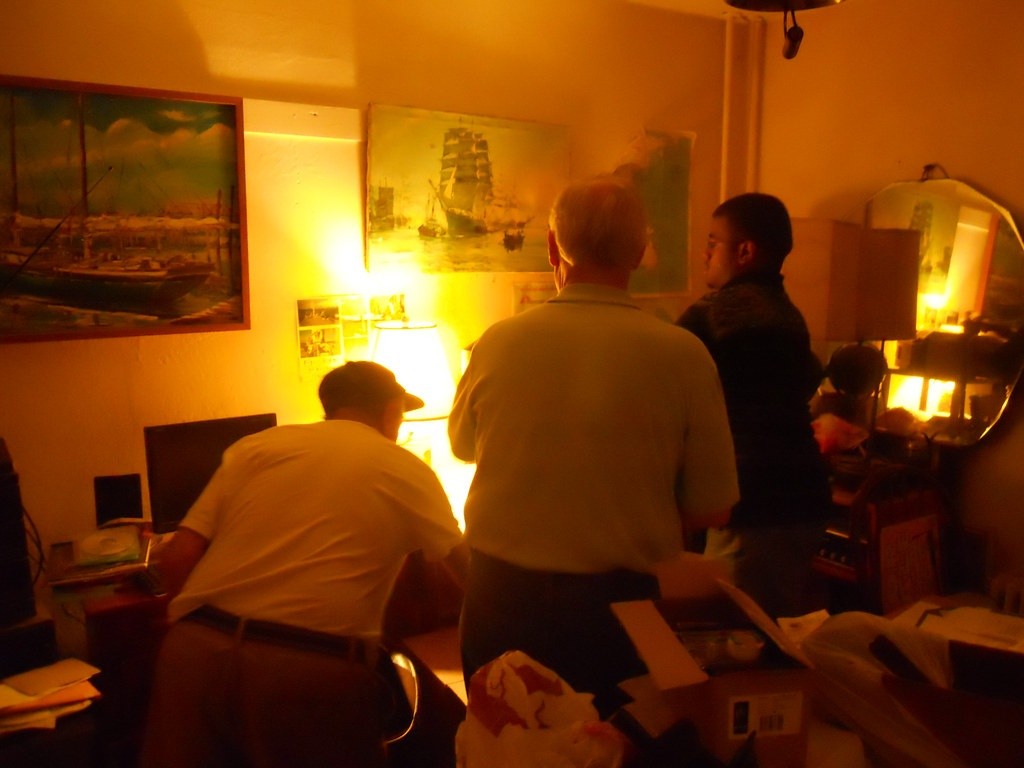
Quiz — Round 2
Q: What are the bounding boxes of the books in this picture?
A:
[47,538,152,587]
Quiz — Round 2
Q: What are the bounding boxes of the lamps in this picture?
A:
[341,296,456,422]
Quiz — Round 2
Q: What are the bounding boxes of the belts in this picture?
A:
[185,604,365,662]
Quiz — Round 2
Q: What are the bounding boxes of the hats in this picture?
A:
[318,361,424,412]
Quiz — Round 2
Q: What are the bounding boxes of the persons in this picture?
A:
[675,192,830,673]
[140,363,467,768]
[450,185,740,768]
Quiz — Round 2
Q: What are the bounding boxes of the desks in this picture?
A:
[880,370,991,440]
[816,466,943,622]
[46,532,192,733]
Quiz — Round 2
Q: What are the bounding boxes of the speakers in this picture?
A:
[93,474,144,527]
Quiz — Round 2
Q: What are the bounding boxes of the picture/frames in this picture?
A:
[864,187,961,296]
[364,101,570,272]
[605,125,698,297]
[0,73,252,345]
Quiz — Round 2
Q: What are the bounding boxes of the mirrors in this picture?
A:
[824,177,1024,449]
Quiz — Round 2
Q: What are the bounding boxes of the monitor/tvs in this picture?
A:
[144,413,277,535]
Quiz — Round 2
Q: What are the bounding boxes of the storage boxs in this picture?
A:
[610,560,815,768]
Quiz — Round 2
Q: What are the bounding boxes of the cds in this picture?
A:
[83,528,136,557]
